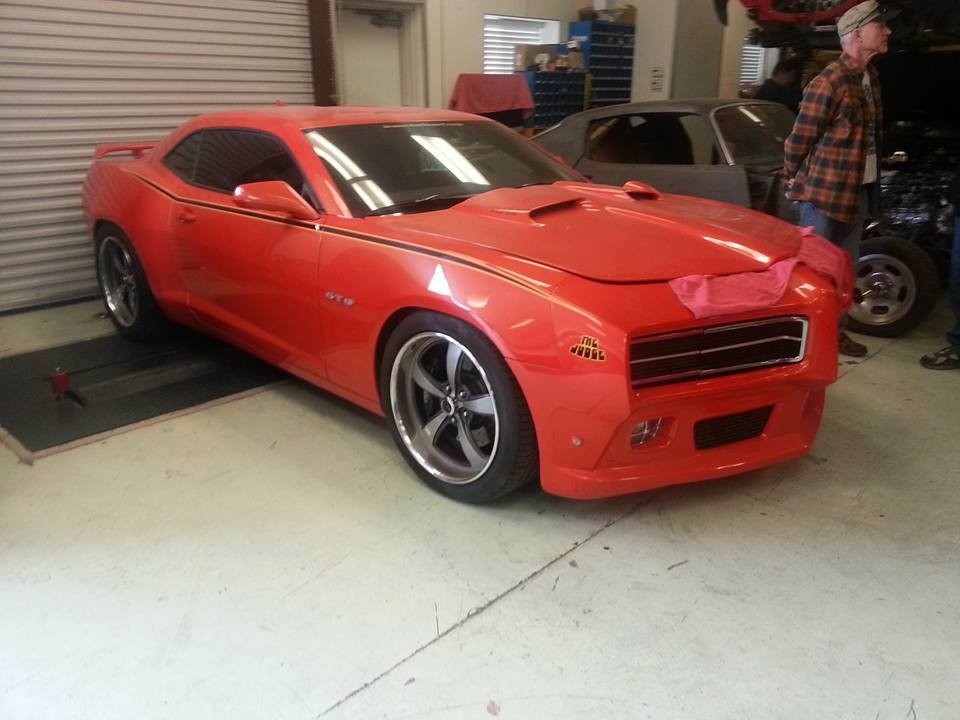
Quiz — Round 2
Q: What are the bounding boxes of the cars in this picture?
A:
[519,94,958,337]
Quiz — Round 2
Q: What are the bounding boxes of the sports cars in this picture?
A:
[84,107,854,501]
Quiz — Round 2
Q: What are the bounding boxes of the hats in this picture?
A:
[838,0,904,37]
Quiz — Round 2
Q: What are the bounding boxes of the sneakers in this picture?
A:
[920,345,960,370]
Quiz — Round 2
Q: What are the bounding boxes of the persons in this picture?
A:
[779,0,893,358]
[754,60,799,118]
[920,211,960,370]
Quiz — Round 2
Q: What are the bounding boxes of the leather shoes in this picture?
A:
[837,332,867,356]
